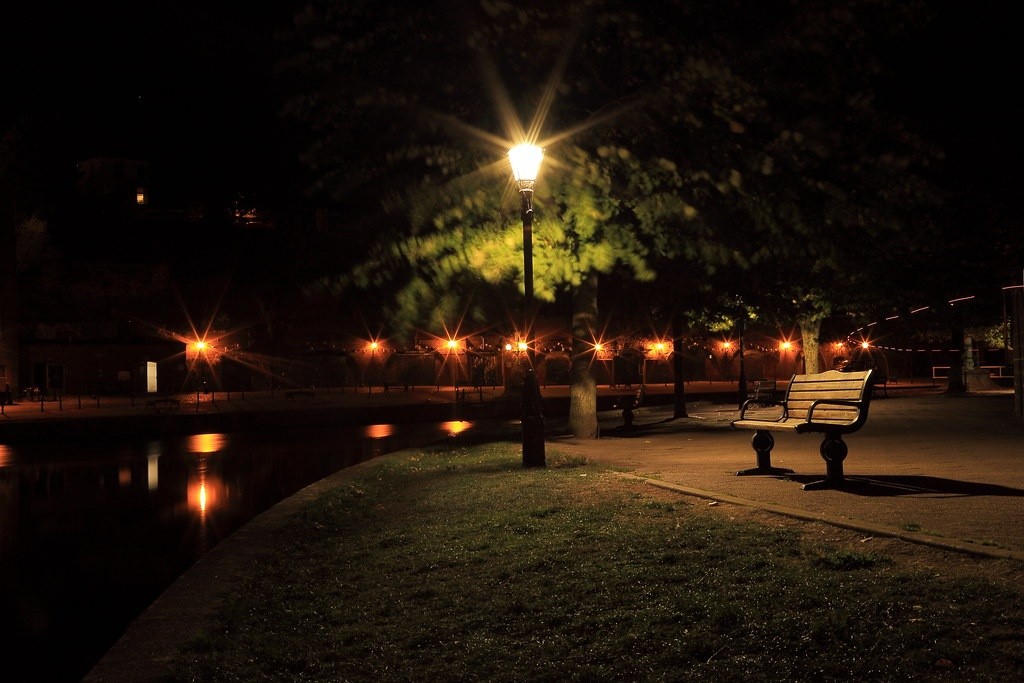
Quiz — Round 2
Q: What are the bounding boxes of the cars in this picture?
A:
[618,394,639,410]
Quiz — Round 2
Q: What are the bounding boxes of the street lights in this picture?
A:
[732,318,748,409]
[862,342,869,371]
[709,355,712,384]
[507,141,545,467]
[198,342,204,387]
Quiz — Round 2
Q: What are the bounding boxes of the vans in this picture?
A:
[746,377,776,392]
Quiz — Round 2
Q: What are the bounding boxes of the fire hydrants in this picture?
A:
[616,405,639,431]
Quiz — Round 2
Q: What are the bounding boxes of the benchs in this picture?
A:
[731,369,874,491]
[748,380,776,406]
